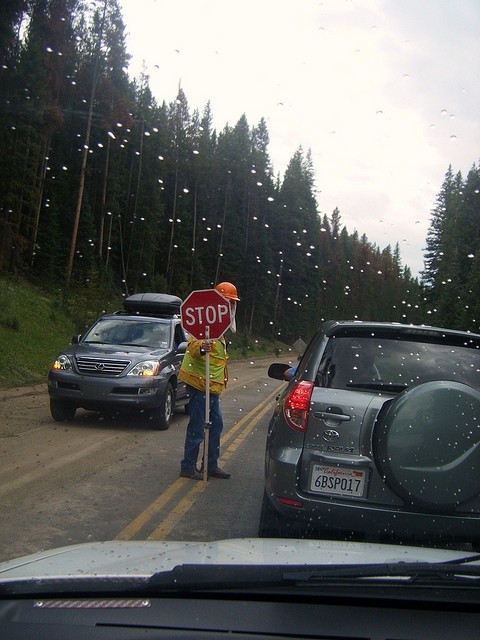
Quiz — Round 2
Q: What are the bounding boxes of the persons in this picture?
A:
[284,366,298,381]
[176,281,241,481]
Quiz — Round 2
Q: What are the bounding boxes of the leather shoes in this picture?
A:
[180,468,209,480]
[200,466,230,479]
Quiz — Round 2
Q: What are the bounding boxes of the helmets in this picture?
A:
[214,282,241,301]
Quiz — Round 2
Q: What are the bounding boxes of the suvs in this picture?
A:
[48,293,190,431]
[259,319,480,547]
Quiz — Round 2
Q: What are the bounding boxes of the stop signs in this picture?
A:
[180,288,233,483]
